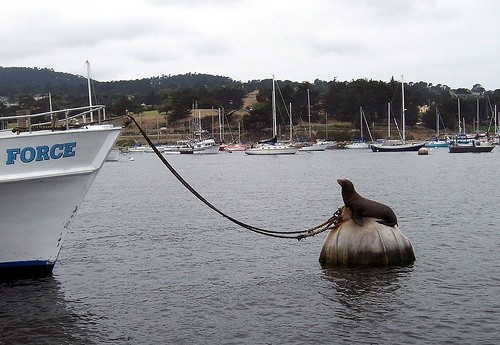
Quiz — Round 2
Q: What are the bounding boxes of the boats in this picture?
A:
[369,138,427,152]
[298,143,328,151]
[128,135,251,154]
[0,103,132,280]
[245,141,305,155]
[316,135,453,149]
[448,131,500,152]
